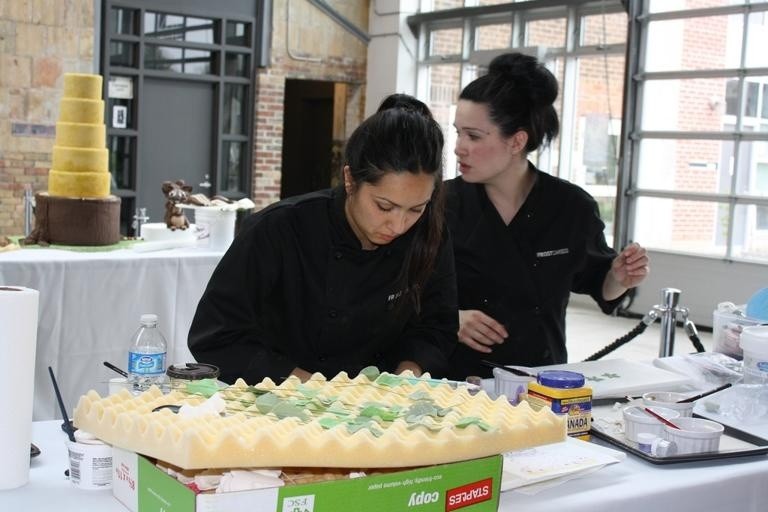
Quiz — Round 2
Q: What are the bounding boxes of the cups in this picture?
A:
[108,374,135,397]
[492,363,536,400]
[67,431,113,487]
[620,389,724,457]
[167,362,222,392]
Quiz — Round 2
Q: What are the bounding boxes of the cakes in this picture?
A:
[19,73,121,245]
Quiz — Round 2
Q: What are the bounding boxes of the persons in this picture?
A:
[184,91,465,384]
[439,52,651,378]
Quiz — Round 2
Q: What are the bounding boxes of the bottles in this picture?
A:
[130,313,167,388]
[528,370,591,442]
